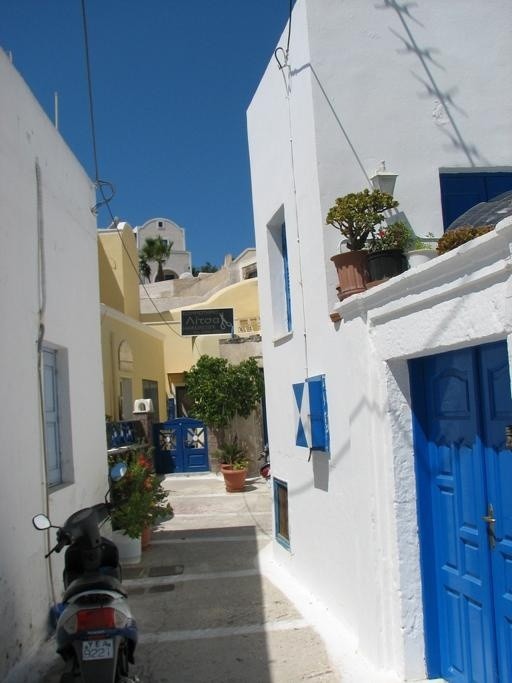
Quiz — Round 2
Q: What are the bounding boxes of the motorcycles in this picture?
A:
[32,462,138,683]
[257,443,270,488]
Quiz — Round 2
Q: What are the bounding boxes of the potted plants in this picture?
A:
[112,447,174,546]
[111,491,149,565]
[367,220,412,281]
[326,188,399,300]
[402,232,438,267]
[214,432,256,492]
